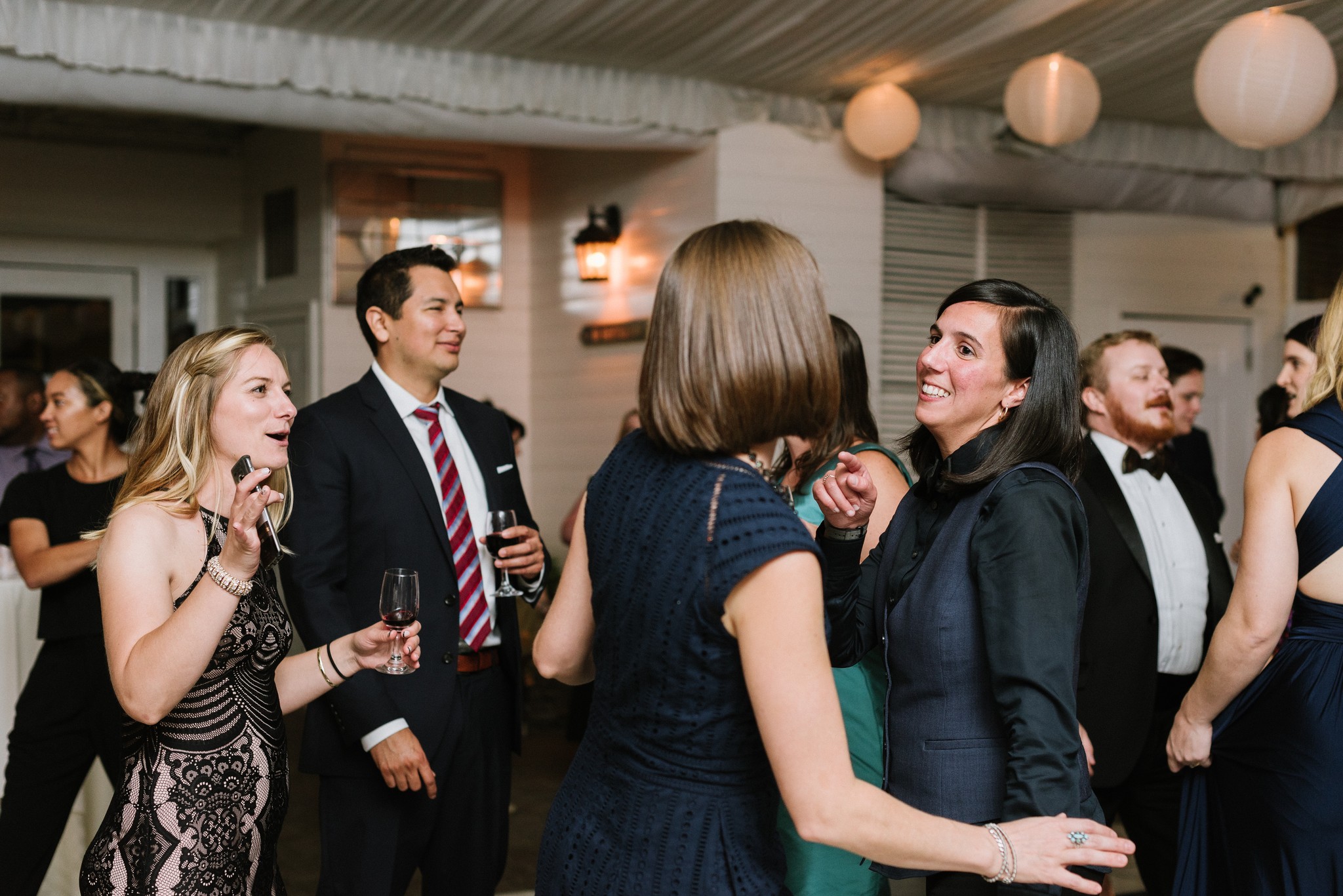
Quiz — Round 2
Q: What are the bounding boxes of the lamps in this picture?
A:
[1194,8,1337,150]
[1005,52,1101,148]
[573,203,622,283]
[845,84,921,160]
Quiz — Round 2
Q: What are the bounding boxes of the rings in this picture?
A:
[1066,830,1089,847]
[822,474,836,486]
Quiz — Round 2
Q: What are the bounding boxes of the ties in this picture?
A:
[21,446,42,475]
[414,401,493,650]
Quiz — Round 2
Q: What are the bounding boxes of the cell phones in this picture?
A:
[231,455,283,572]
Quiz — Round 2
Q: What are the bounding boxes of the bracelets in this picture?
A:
[316,640,350,689]
[982,821,1017,885]
[205,556,254,597]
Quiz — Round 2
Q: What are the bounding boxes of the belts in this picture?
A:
[457,649,503,673]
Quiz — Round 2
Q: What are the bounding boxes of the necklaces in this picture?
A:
[744,450,800,518]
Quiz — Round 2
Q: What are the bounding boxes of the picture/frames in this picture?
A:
[332,156,507,310]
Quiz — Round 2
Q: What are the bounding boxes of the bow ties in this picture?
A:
[1121,444,1168,482]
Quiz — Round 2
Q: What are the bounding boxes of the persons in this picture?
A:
[78,325,423,895]
[279,244,553,894]
[1274,312,1325,418]
[1165,265,1343,895]
[766,314,918,896]
[479,395,527,817]
[531,213,1138,896]
[0,357,76,548]
[1253,381,1294,442]
[1066,327,1236,896]
[1158,343,1227,550]
[560,407,643,549]
[811,276,1116,896]
[0,352,163,896]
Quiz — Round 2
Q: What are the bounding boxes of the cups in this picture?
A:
[375,568,418,675]
[486,510,523,597]
